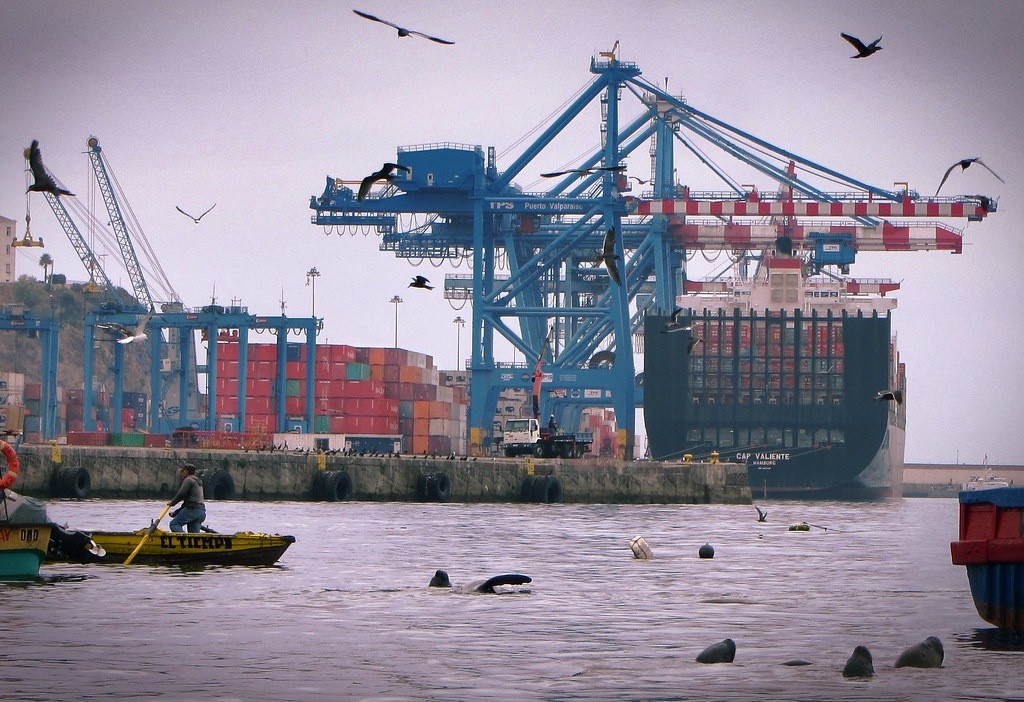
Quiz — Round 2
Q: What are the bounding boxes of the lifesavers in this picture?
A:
[419,470,451,501]
[522,474,561,504]
[312,470,353,502]
[195,467,236,501]
[54,466,91,498]
[0,438,20,491]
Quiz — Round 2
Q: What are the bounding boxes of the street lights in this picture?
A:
[453,317,467,370]
[388,295,405,347]
[307,266,322,317]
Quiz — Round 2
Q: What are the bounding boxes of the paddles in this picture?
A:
[168,511,219,534]
[123,504,171,566]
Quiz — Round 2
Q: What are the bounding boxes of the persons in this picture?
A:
[549,415,557,433]
[167,464,206,533]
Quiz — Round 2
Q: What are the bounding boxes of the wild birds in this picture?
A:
[406,275,435,291]
[176,203,216,223]
[540,167,679,288]
[931,157,1006,202]
[24,139,77,197]
[755,507,768,523]
[357,163,411,203]
[657,309,707,356]
[840,32,885,59]
[352,9,456,45]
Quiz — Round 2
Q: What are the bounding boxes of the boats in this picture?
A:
[948,465,1024,638]
[642,297,903,501]
[0,439,56,584]
[65,525,297,567]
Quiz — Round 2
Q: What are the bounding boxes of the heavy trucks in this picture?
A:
[500,418,594,460]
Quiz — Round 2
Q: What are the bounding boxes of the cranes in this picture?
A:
[10,132,159,313]
[308,34,998,456]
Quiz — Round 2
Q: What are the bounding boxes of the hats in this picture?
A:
[550,414,554,417]
[176,464,196,472]
